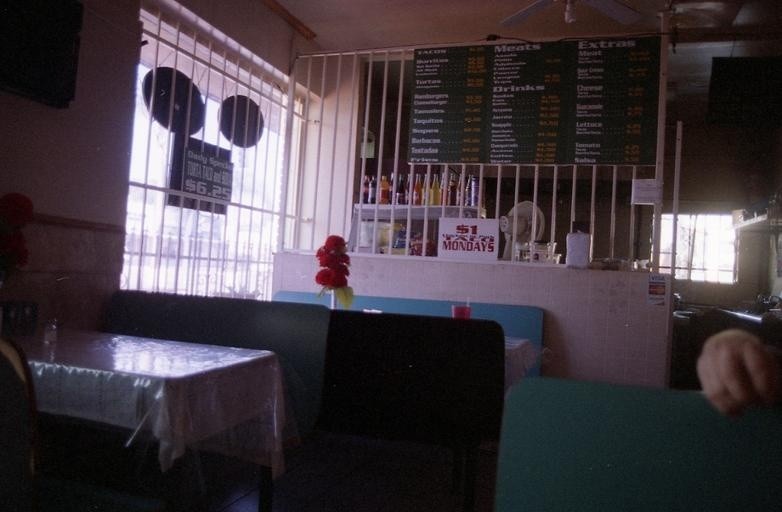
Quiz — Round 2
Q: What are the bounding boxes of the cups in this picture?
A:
[514,240,530,261]
[449,295,472,319]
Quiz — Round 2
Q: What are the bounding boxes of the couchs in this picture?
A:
[94,290,331,511]
[272,290,544,398]
[495,374,782,512]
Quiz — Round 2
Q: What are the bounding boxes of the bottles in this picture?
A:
[42,316,62,347]
[361,172,473,206]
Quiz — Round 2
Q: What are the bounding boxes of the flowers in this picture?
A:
[11,326,286,512]
[0,192,34,288]
[314,235,354,309]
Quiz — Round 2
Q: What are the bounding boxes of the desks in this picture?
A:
[505,337,531,381]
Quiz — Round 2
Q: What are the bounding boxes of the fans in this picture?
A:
[499,0,647,29]
[499,201,545,260]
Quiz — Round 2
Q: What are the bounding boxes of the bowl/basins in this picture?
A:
[532,240,562,264]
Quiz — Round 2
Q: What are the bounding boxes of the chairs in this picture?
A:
[672,309,708,390]
[0,334,43,512]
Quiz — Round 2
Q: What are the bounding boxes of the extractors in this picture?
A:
[730,200,782,235]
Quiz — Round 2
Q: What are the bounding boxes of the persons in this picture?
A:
[669,311,782,419]
